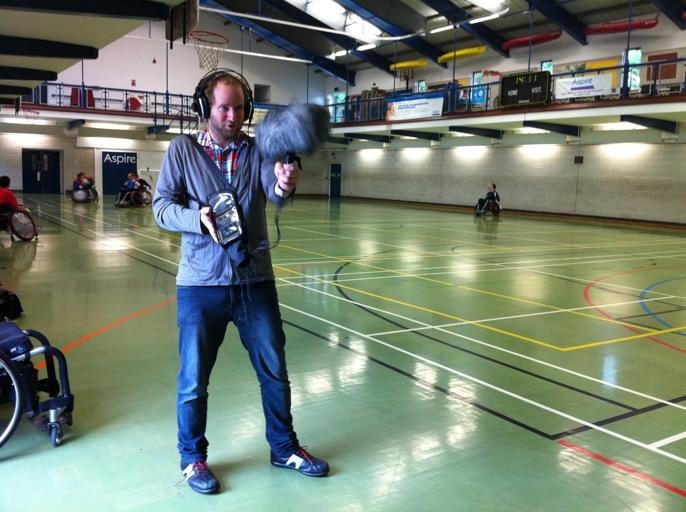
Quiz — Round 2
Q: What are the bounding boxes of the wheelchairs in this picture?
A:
[0,266,23,320]
[115,178,151,210]
[0,320,75,448]
[474,196,501,218]
[0,202,39,243]
[71,181,101,204]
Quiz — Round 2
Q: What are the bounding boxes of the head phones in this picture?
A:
[192,68,253,121]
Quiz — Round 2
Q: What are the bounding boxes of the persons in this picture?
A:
[0,176,32,214]
[118,172,145,208]
[73,171,98,198]
[152,73,330,493]
[134,173,151,189]
[476,184,500,214]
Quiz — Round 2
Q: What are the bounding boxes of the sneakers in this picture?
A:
[271,448,329,477]
[181,460,221,494]
